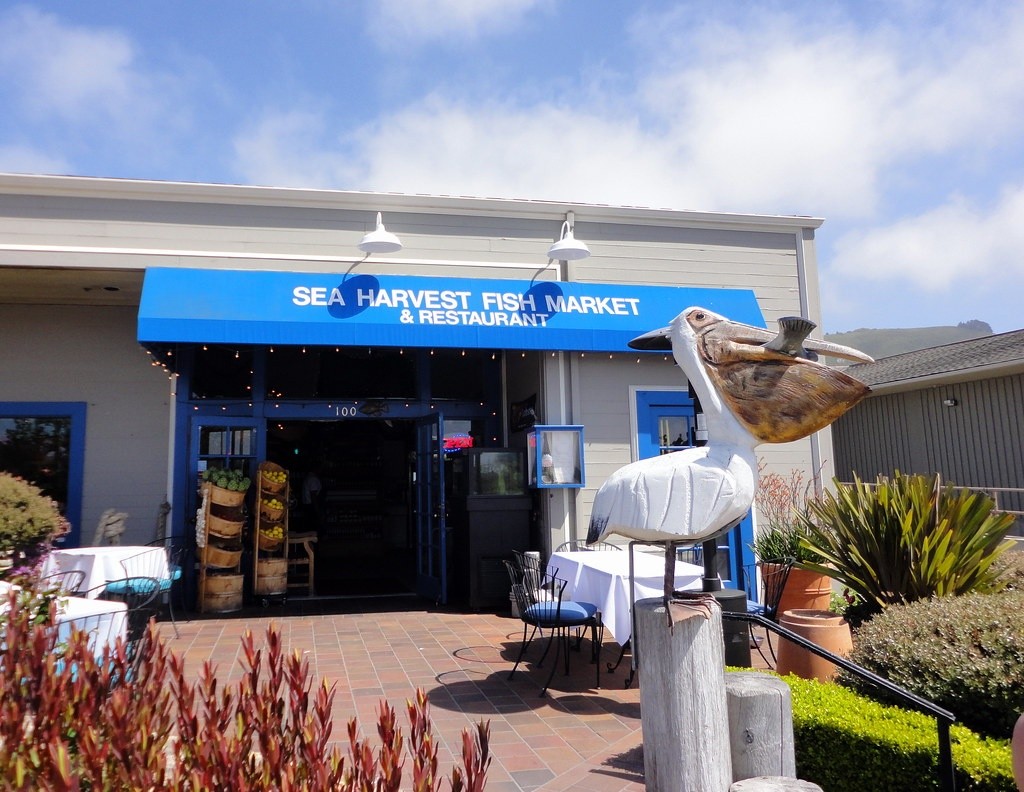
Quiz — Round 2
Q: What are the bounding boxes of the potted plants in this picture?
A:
[745,455,835,622]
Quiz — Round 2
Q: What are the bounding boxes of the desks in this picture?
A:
[288,531,318,596]
[541,552,705,690]
[0,593,128,660]
[36,546,171,600]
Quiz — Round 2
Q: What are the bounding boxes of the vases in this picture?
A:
[533,424,586,488]
[777,609,854,684]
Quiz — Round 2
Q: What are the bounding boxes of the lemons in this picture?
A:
[263,498,283,509]
[260,526,284,539]
[262,471,287,483]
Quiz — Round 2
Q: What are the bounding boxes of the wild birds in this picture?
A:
[586,305,874,634]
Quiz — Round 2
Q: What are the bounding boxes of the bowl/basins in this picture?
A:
[750,635,764,648]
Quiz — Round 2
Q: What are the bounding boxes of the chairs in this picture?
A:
[741,556,796,669]
[675,537,720,566]
[501,558,601,699]
[555,539,625,638]
[45,608,164,689]
[512,550,598,669]
[104,542,187,640]
[40,571,86,597]
[76,576,161,664]
[146,534,194,622]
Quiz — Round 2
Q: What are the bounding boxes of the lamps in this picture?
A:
[546,220,591,261]
[357,211,403,254]
[944,400,957,407]
